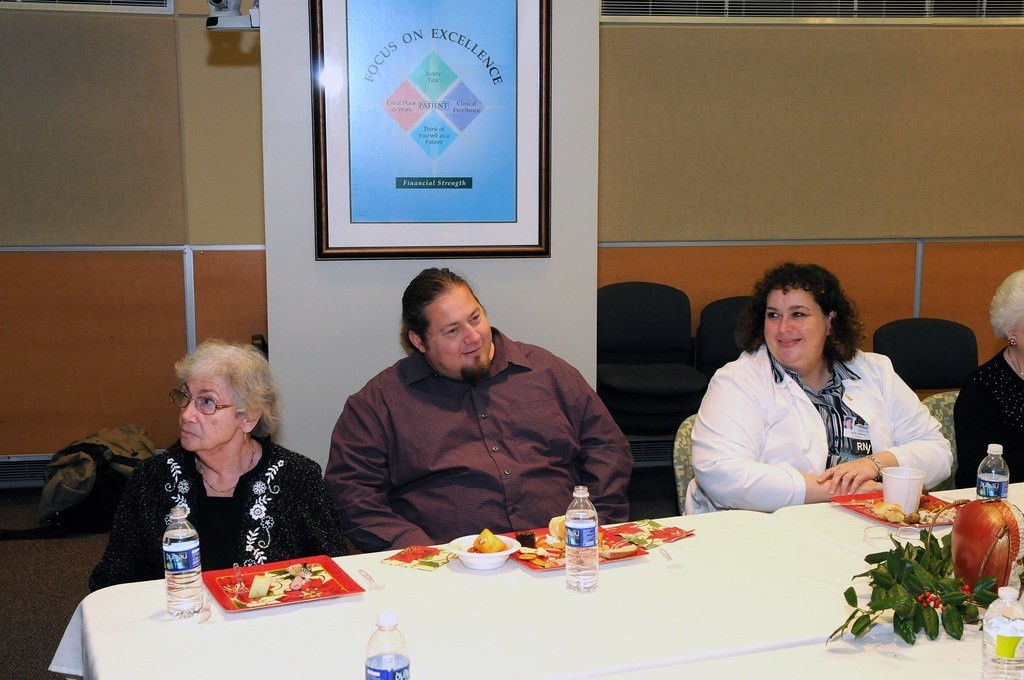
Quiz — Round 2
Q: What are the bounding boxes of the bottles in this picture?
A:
[162,507,203,618]
[365,610,409,680]
[975,444,1009,502]
[565,486,599,592]
[981,587,1024,680]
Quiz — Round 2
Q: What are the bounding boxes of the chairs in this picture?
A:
[597,281,979,516]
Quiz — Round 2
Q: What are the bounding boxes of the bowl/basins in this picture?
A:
[446,534,521,571]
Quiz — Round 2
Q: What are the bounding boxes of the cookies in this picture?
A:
[519,541,550,567]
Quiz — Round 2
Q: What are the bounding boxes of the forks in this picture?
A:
[233,562,249,594]
[658,548,683,569]
[360,570,384,591]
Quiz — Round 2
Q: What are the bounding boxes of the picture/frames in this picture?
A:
[308,0,553,260]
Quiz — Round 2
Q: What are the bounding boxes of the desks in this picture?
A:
[47,482,1024,680]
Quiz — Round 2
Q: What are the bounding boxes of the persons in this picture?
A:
[89,338,350,591]
[684,262,953,514]
[325,268,633,552]
[953,269,1023,489]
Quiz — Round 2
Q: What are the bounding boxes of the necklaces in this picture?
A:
[199,441,255,493]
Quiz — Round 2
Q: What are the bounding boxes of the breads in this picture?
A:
[873,502,904,524]
[602,544,638,560]
[549,515,567,541]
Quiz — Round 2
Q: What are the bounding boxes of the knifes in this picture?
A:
[198,593,212,624]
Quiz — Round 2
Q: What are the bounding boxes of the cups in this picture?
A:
[882,467,927,516]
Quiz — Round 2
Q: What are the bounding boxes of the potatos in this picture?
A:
[473,527,506,553]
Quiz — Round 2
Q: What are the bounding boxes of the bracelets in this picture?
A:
[863,455,883,482]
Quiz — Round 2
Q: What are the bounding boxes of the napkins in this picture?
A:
[604,520,695,550]
[381,546,458,571]
